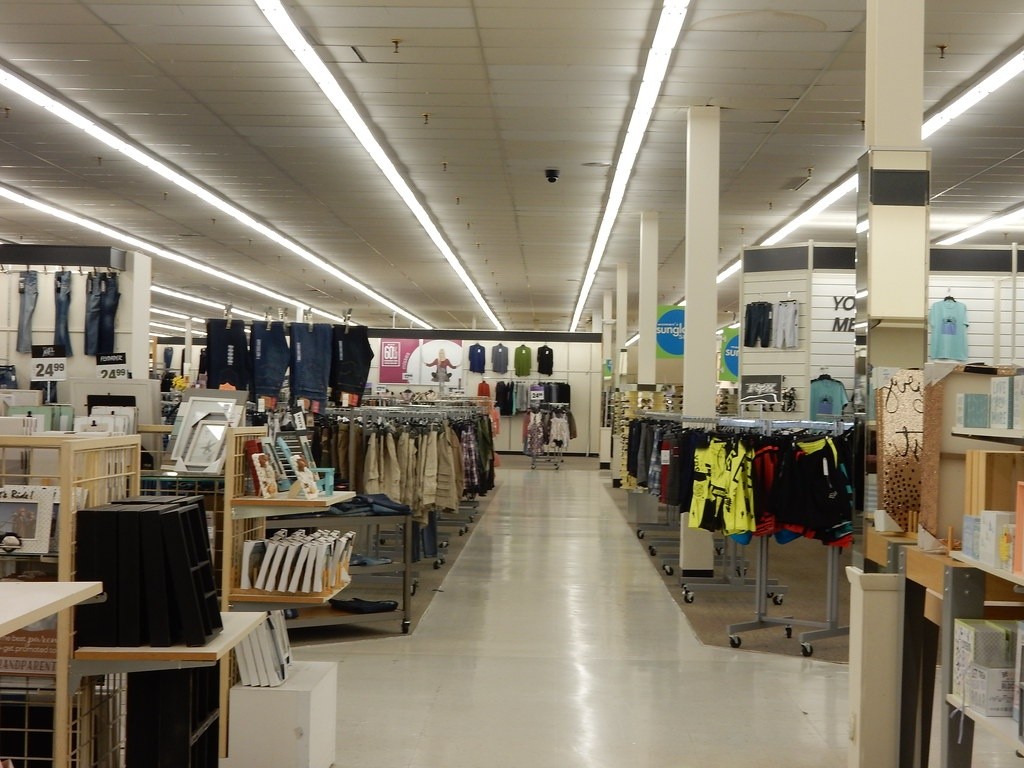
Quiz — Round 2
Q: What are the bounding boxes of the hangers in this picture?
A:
[780,292,796,302]
[474,343,480,346]
[944,291,955,302]
[820,366,830,375]
[481,377,566,384]
[499,343,502,346]
[544,344,547,347]
[328,395,496,432]
[751,291,767,305]
[521,344,525,346]
[645,412,843,434]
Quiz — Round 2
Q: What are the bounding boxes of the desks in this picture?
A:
[240,527,356,594]
[56,611,268,768]
[221,660,336,768]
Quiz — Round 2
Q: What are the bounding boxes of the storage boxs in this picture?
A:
[952,618,1017,716]
[233,612,293,687]
[955,392,991,428]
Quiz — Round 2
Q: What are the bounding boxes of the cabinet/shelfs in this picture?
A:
[897,545,1024,768]
[0,433,140,768]
[139,423,413,634]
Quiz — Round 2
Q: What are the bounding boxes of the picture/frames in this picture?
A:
[0,580,103,639]
[185,420,229,466]
[74,407,138,433]
[0,486,88,553]
